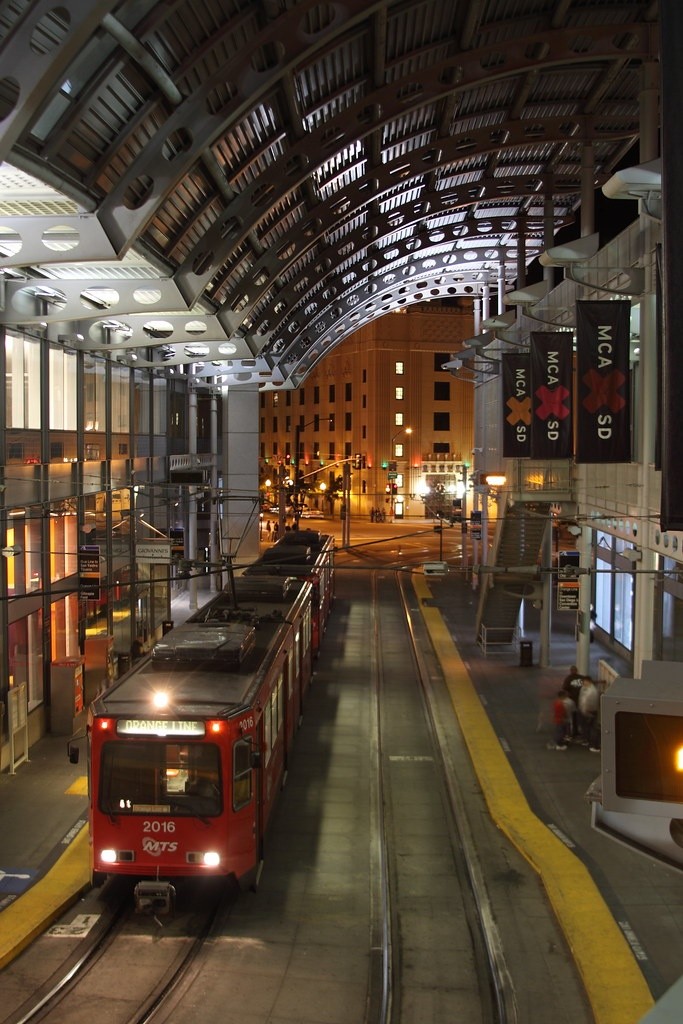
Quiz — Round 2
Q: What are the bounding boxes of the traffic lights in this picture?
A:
[392,484,397,495]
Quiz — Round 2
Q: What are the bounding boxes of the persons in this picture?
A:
[266,521,271,541]
[273,521,298,536]
[369,507,386,523]
[130,635,148,663]
[388,507,396,523]
[552,665,601,754]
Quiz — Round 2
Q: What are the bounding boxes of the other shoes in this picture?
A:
[561,745,567,750]
[555,746,561,750]
[588,747,601,752]
[581,742,589,746]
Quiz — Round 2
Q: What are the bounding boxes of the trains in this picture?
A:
[67,529,335,927]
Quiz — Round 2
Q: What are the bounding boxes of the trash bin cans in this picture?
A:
[161,620,174,637]
[519,640,533,667]
[117,652,130,679]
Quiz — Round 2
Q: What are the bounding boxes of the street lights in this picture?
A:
[481,474,507,567]
[265,479,271,501]
[389,428,412,523]
[260,513,264,541]
[320,482,326,512]
